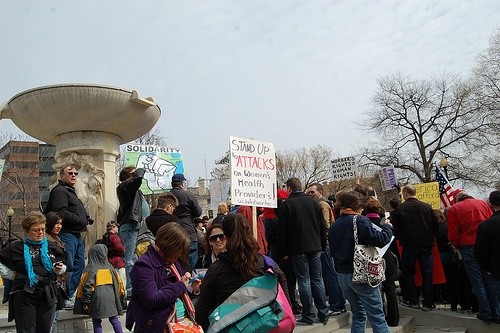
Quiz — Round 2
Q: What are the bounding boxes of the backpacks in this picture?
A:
[206,254,296,333]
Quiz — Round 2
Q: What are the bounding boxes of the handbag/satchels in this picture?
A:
[352,245,386,288]
[164,317,204,333]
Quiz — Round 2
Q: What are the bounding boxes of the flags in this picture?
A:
[436,166,457,211]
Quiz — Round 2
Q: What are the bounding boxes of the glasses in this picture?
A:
[64,172,78,176]
[29,228,46,232]
[210,234,226,242]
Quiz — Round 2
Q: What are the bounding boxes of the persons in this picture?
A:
[306,182,347,316]
[475,191,500,324]
[395,183,437,312]
[168,173,203,273]
[431,207,478,313]
[145,191,193,278]
[47,163,94,310]
[189,222,230,298]
[328,192,395,333]
[102,219,131,312]
[0,211,68,333]
[352,185,400,327]
[116,165,149,300]
[126,221,202,333]
[72,244,127,333]
[278,177,330,325]
[193,211,292,333]
[194,181,303,318]
[447,191,500,323]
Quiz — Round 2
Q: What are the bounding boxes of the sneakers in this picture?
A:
[61,296,76,310]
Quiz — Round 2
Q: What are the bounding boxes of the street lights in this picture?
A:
[6,206,15,245]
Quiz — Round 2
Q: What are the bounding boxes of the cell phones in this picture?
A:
[53,261,62,267]
[188,273,206,284]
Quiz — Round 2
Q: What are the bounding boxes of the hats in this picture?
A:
[277,189,288,199]
[172,173,187,183]
[106,220,120,227]
[453,189,465,202]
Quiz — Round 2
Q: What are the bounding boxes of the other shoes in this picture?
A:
[419,302,436,311]
[477,313,500,324]
[399,297,419,309]
[291,305,303,316]
[296,318,314,325]
[327,309,346,315]
[316,313,329,323]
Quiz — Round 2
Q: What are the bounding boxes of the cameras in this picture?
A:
[86,215,94,227]
